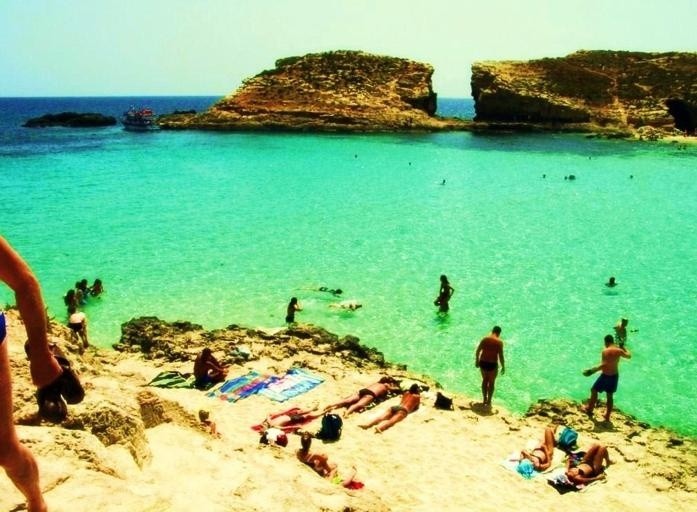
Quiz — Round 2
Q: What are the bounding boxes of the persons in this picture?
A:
[607,276,618,286]
[615,316,630,345]
[262,374,427,474]
[194,348,227,383]
[518,425,559,480]
[0,237,64,512]
[583,335,632,421]
[556,444,614,486]
[476,326,505,404]
[317,286,343,299]
[286,296,303,323]
[435,276,454,313]
[330,303,363,310]
[61,277,104,348]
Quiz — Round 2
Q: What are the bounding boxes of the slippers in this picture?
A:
[22,338,86,425]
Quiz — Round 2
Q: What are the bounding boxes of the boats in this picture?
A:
[121,107,161,132]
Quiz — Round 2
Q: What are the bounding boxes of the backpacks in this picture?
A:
[559,427,579,448]
[316,412,343,444]
